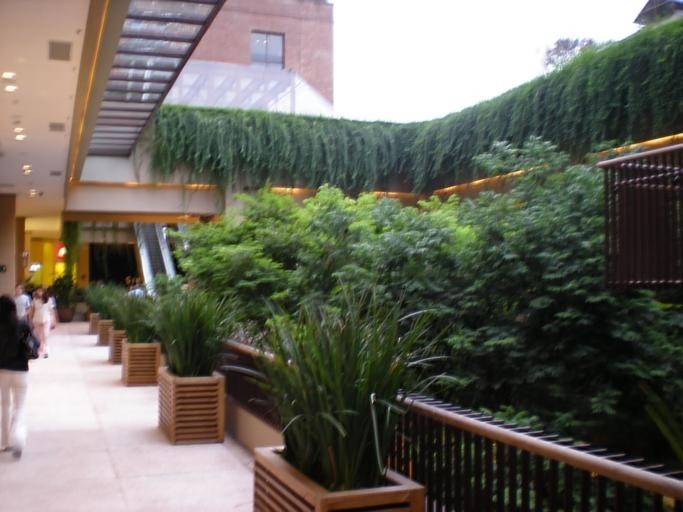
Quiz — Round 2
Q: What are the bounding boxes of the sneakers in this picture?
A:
[2,443,21,459]
[44,354,48,358]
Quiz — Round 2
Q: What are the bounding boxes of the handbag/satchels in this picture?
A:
[16,331,41,359]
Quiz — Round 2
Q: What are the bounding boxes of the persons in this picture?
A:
[11,283,30,324]
[0,294,40,459]
[28,287,54,359]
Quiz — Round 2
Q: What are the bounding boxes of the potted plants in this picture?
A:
[89,286,102,334]
[137,285,246,447]
[102,285,140,366]
[96,286,123,346]
[52,275,86,322]
[118,286,162,386]
[251,275,455,509]
